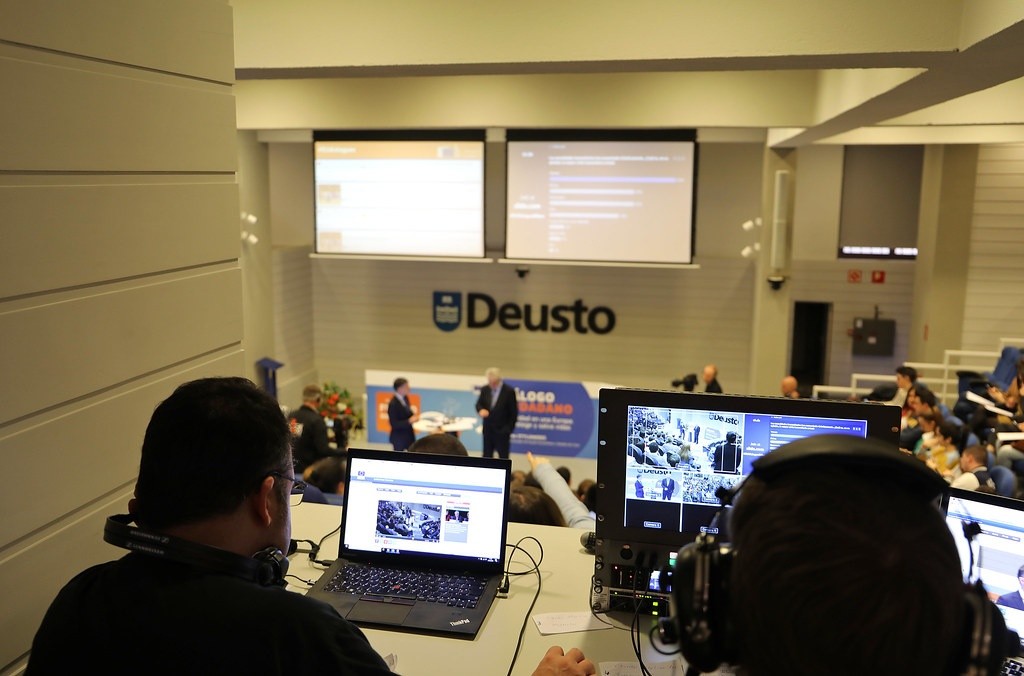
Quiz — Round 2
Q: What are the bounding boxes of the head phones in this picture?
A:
[104,499,290,588]
[668,431,1022,676]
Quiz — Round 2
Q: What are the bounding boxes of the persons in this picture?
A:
[387,378,420,451]
[22,377,595,676]
[407,434,468,455]
[996,566,1024,610]
[781,376,799,398]
[287,385,347,473]
[843,350,1024,499]
[506,471,567,526]
[635,419,742,500]
[555,467,596,521]
[703,365,722,393]
[378,500,469,542]
[727,436,966,676]
[474,367,518,459]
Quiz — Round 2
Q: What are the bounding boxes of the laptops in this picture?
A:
[938,485,1024,676]
[305,447,512,641]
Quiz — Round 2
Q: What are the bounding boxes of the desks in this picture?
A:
[282,500,690,676]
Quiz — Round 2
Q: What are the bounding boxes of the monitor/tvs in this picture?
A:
[595,388,902,550]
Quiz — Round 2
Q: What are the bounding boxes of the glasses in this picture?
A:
[260,469,308,506]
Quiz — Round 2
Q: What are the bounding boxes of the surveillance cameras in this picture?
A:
[516,267,530,278]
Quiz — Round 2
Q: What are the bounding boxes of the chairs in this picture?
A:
[919,346,1024,498]
